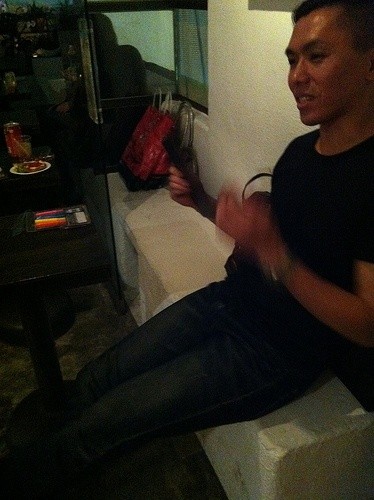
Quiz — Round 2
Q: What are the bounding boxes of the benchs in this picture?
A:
[99,171,374,500]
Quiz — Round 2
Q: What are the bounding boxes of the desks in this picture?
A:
[0,55,128,452]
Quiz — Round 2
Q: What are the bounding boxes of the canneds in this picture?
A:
[4,122,23,159]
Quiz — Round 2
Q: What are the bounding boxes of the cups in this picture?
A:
[12,134,32,158]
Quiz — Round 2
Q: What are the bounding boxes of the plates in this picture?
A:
[9,161,52,175]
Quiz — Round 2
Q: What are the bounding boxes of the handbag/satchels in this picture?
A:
[168,102,193,166]
[224,169,277,274]
[120,87,174,182]
